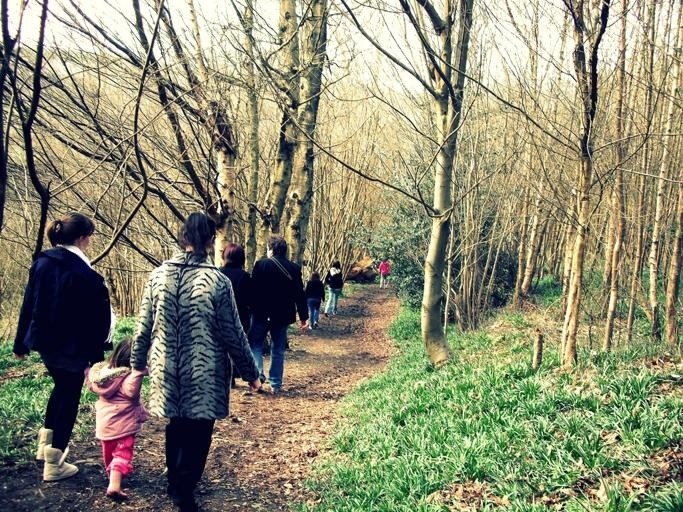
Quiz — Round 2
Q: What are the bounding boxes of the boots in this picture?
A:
[42,442,78,480]
[35,426,54,460]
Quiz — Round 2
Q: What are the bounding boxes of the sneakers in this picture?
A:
[105,487,130,500]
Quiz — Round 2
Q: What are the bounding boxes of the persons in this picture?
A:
[216,242,252,389]
[83,335,150,498]
[305,272,326,330]
[246,236,309,393]
[379,258,391,289]
[130,212,262,512]
[324,260,343,315]
[11,213,116,481]
